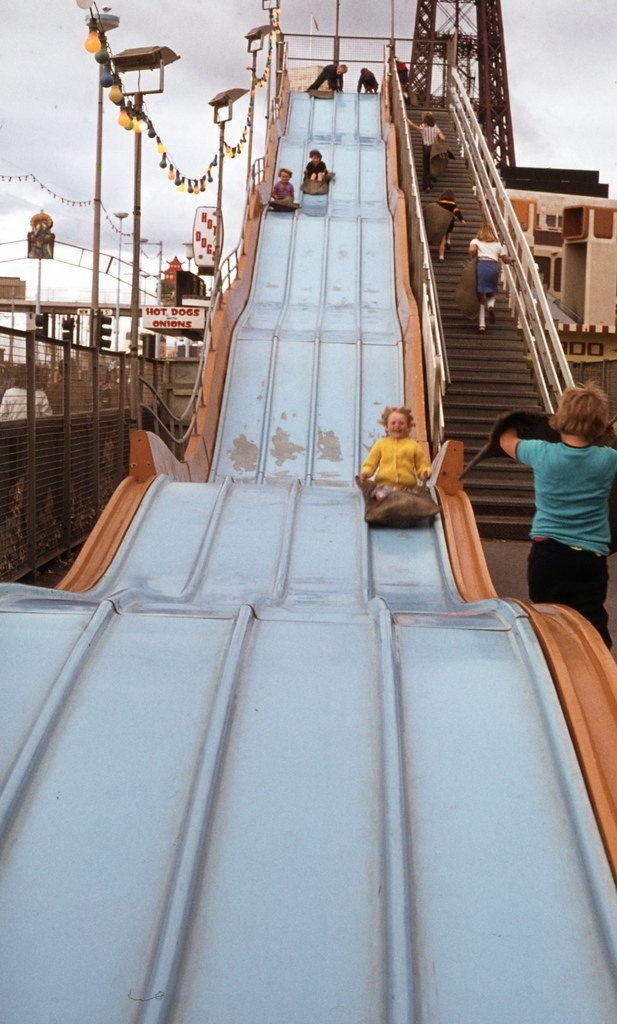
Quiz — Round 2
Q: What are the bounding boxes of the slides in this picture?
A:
[0,89,617,1022]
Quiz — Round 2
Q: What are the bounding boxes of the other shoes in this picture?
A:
[447,242,452,251]
[438,257,445,263]
[488,307,496,326]
[478,328,484,333]
[426,188,431,194]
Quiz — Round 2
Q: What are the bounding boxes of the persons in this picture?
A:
[358,68,378,94]
[47,359,91,412]
[307,65,347,93]
[500,384,617,648]
[0,366,53,421]
[469,223,516,333]
[304,150,328,182]
[98,368,119,468]
[387,56,410,105]
[361,405,432,500]
[438,190,465,262]
[272,168,294,201]
[406,115,446,193]
